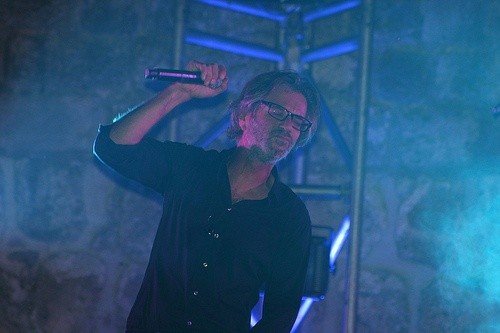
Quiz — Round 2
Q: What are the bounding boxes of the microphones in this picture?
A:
[145,67,223,88]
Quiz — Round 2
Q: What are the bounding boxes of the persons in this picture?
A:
[92,61,322,333]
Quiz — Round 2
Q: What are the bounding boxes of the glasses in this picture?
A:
[260,100,313,132]
